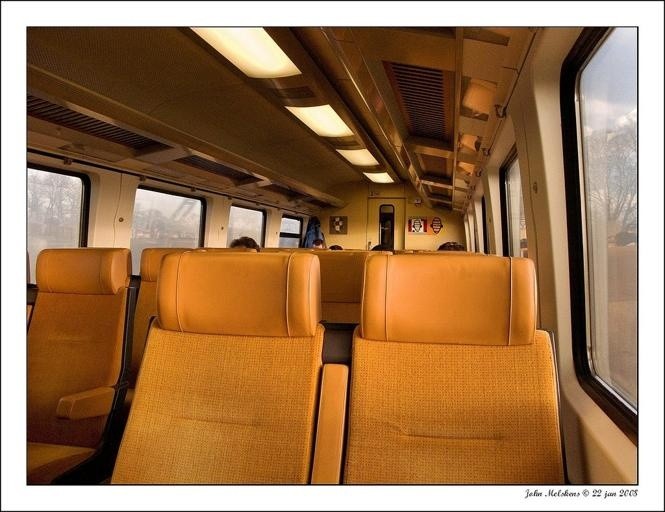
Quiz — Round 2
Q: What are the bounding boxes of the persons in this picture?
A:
[312,239,325,248]
[329,244,343,250]
[437,241,465,251]
[229,236,261,252]
[371,244,395,253]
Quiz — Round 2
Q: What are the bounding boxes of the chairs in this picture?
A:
[26,246,570,485]
